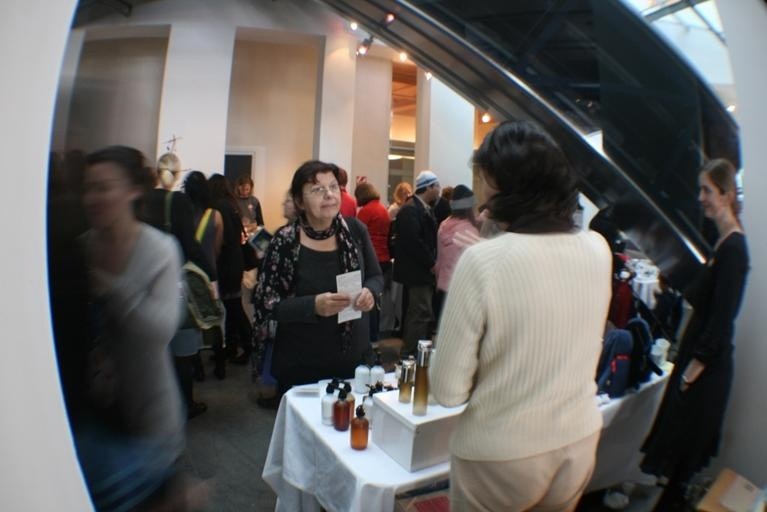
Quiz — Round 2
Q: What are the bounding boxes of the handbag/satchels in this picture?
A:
[180,261,226,330]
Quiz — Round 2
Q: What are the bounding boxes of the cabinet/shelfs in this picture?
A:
[263,363,672,512]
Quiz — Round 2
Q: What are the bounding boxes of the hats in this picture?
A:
[450,185,475,210]
[416,170,439,189]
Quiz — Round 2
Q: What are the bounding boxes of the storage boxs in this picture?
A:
[696,470,766,511]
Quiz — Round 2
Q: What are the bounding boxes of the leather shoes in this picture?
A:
[258,395,278,410]
[188,400,207,418]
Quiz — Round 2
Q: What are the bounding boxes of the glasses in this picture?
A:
[304,184,338,196]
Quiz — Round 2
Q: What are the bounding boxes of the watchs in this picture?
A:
[680,376,691,388]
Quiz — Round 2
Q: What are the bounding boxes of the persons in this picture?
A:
[651,266,680,342]
[179,171,225,380]
[587,198,639,251]
[253,160,385,391]
[234,177,263,263]
[610,251,636,329]
[256,185,297,408]
[335,166,359,218]
[354,176,393,343]
[208,174,252,362]
[388,183,415,222]
[436,188,454,228]
[429,120,612,512]
[431,185,488,338]
[389,170,440,358]
[132,153,207,421]
[640,159,750,511]
[75,146,182,511]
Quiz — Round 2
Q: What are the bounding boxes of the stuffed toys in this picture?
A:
[597,329,633,397]
[627,320,663,388]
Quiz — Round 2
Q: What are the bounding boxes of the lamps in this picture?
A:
[360,35,376,56]
